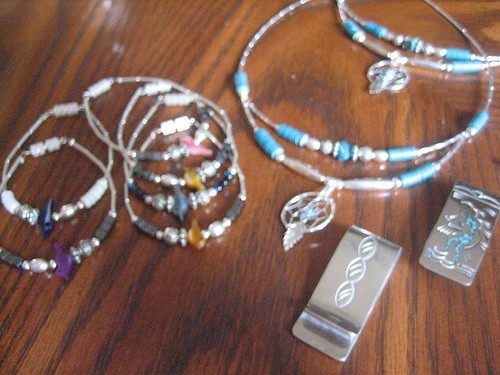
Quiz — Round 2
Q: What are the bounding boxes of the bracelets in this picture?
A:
[1,70,247,283]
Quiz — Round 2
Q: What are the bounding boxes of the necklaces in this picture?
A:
[233,1,496,254]
[337,0,500,95]
